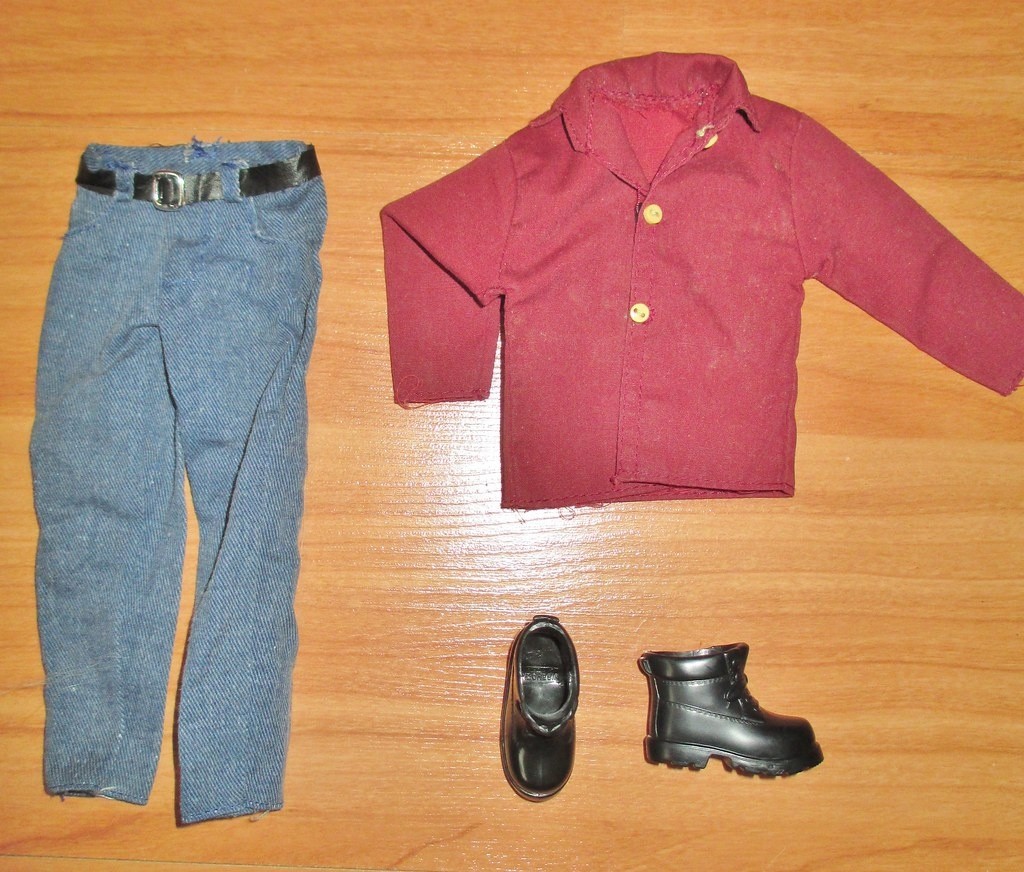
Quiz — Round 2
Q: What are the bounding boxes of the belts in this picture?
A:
[74,144,326,210]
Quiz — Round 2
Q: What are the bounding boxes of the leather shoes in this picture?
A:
[642,643,828,777]
[500,616,582,800]
